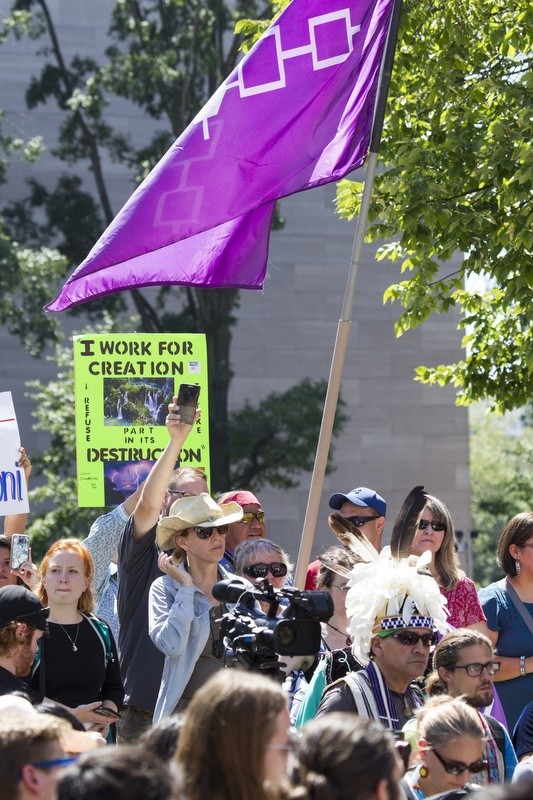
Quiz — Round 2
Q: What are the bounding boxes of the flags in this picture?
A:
[44,0,403,310]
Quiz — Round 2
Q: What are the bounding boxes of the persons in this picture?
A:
[31,538,124,748]
[0,453,533,800]
[79,478,146,660]
[478,510,533,732]
[300,486,387,594]
[117,396,211,741]
[234,537,330,666]
[149,493,264,723]
[178,667,297,800]
[413,495,506,733]
[216,490,264,576]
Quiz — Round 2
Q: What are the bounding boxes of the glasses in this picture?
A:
[169,489,197,498]
[389,630,438,647]
[349,515,385,527]
[432,749,488,775]
[244,562,288,579]
[330,582,349,594]
[418,519,446,531]
[14,605,51,621]
[194,524,228,540]
[241,510,266,524]
[454,661,502,678]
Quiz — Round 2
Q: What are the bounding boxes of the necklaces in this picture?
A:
[388,682,413,719]
[48,613,80,651]
[185,562,225,659]
[326,622,353,646]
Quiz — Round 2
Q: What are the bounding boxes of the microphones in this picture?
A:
[211,579,280,603]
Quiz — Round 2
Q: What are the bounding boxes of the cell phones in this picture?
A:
[175,384,200,424]
[93,706,122,719]
[10,534,31,569]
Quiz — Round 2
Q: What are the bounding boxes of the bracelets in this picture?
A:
[520,656,526,677]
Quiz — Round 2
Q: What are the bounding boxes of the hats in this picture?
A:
[218,490,262,508]
[318,484,456,667]
[303,560,322,592]
[0,584,51,631]
[155,492,244,551]
[328,486,387,516]
